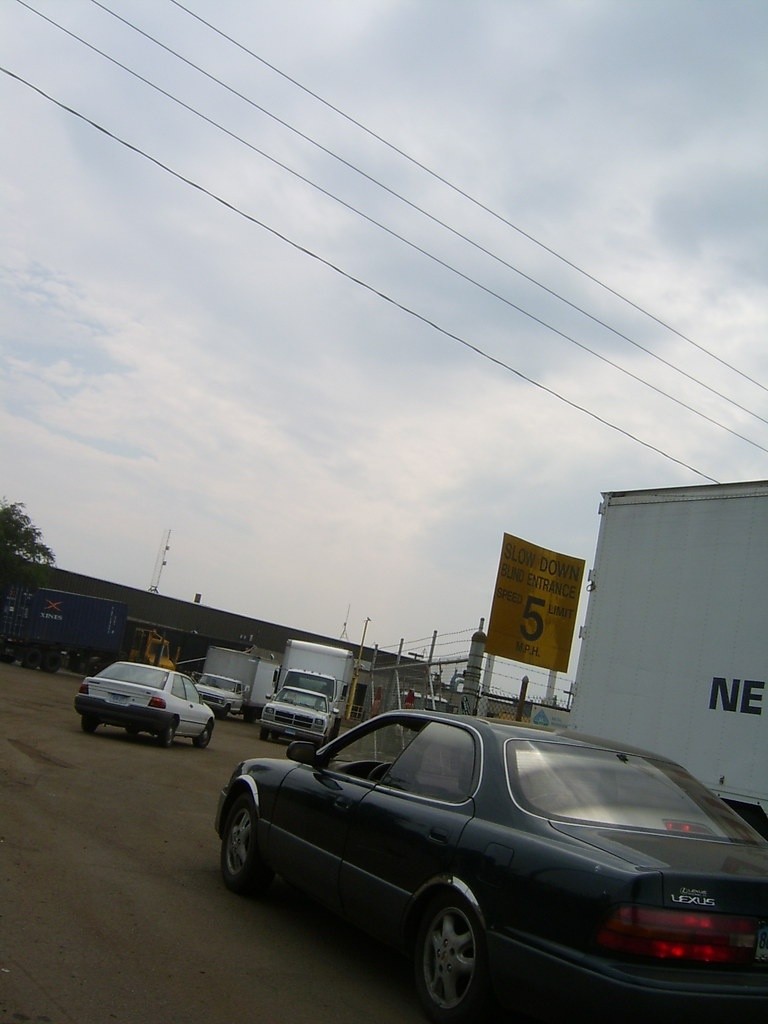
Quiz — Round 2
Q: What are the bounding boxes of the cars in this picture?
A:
[74,661,216,749]
[212,709,768,1022]
[259,685,339,749]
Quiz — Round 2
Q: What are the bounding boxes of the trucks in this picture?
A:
[195,645,276,724]
[277,639,355,720]
[0,586,175,674]
